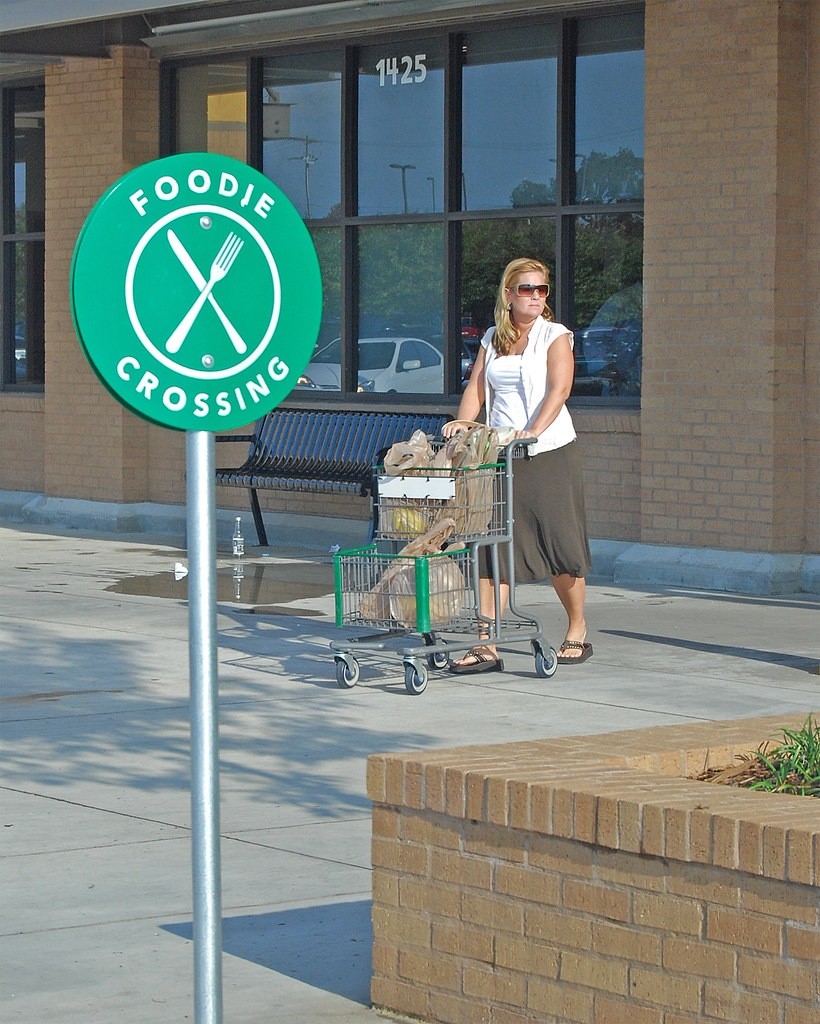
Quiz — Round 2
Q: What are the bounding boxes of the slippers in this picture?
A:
[449,651,504,674]
[556,640,593,664]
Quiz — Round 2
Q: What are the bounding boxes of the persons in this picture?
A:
[582,248,643,398]
[441,257,593,673]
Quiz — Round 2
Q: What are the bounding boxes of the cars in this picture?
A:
[576,329,642,395]
[16,322,26,378]
[295,313,489,395]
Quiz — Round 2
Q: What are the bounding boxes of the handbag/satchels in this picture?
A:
[356,423,502,628]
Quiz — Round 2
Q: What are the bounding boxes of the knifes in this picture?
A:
[167,229,247,354]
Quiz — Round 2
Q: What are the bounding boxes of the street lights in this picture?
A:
[389,163,417,213]
[427,176,435,212]
[576,153,586,200]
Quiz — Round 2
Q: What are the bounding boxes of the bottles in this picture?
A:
[232,564,244,600]
[232,517,245,561]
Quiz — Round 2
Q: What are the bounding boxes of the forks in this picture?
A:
[166,232,245,354]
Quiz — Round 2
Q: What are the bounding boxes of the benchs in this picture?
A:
[214,406,456,549]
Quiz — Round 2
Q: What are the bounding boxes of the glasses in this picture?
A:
[508,284,550,297]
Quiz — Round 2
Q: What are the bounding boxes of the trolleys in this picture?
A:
[330,427,558,695]
